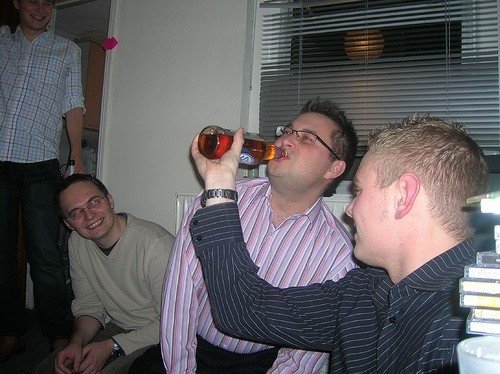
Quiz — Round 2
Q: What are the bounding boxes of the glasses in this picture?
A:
[276,125,340,160]
[62,196,106,220]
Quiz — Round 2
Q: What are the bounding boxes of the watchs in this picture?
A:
[110,337,124,358]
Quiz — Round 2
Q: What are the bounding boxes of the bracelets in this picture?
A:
[198,188,242,208]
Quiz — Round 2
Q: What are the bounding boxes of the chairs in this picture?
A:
[175,193,354,239]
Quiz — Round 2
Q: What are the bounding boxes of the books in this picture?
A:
[458,189,500,334]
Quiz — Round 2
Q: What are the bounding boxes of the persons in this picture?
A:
[160,94,363,374]
[31,174,184,373]
[189,112,495,374]
[0,0,86,362]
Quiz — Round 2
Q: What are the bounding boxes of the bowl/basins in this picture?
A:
[457,336,500,374]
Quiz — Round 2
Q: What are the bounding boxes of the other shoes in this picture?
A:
[0,334,25,362]
[50,337,69,350]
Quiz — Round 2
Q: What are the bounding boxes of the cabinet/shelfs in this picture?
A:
[75,40,105,131]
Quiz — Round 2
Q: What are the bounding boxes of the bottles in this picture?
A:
[197,125,286,165]
[66,159,77,179]
